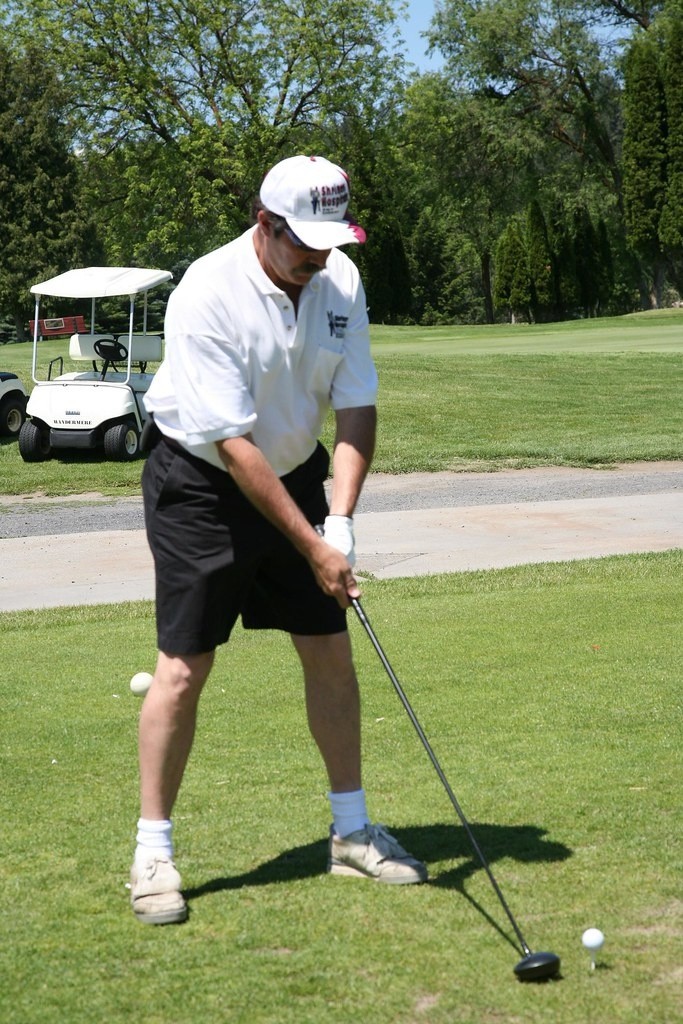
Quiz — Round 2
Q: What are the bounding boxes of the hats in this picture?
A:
[259,155,367,250]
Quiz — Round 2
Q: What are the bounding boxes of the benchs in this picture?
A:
[54,335,162,392]
[29,316,89,342]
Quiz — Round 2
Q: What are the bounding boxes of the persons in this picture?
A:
[131,156,429,926]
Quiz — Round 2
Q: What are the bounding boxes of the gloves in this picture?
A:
[320,511,357,570]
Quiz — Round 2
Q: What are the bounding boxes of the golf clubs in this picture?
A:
[309,521,562,984]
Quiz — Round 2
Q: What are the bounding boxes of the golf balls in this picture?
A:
[580,927,607,950]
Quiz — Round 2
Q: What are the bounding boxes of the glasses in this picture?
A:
[284,228,308,249]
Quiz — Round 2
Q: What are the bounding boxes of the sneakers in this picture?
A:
[130,856,187,926]
[327,822,428,885]
